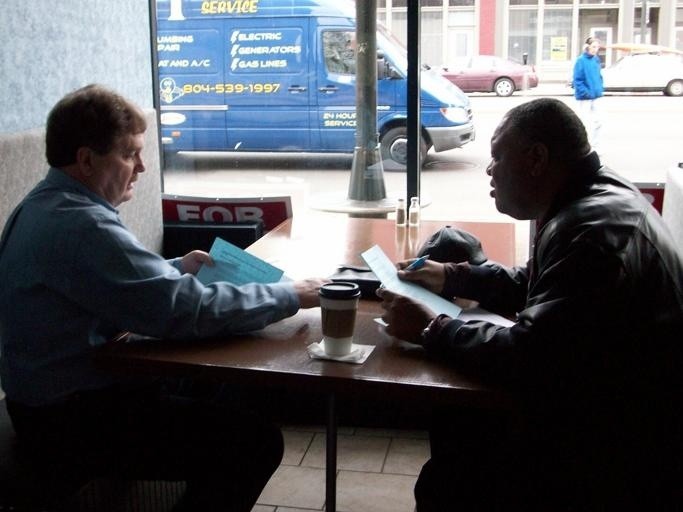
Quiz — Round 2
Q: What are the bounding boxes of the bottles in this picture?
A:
[396,198,406,226]
[408,197,421,226]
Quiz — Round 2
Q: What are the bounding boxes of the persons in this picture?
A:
[375,98,682,511]
[571,37,607,158]
[0,83,338,510]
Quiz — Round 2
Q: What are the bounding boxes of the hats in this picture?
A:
[417,226,487,265]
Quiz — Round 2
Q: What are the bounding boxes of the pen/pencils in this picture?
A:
[380,254,430,289]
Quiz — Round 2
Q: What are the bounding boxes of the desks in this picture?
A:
[112,213,515,507]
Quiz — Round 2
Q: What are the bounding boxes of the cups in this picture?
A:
[318,281,361,357]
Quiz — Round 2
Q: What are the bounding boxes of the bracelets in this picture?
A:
[419,319,437,350]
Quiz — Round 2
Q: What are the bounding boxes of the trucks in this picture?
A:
[154,0,475,173]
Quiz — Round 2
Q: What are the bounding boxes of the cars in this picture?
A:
[437,54,538,98]
[566,53,683,98]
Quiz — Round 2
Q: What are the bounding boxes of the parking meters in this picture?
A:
[522,53,528,96]
